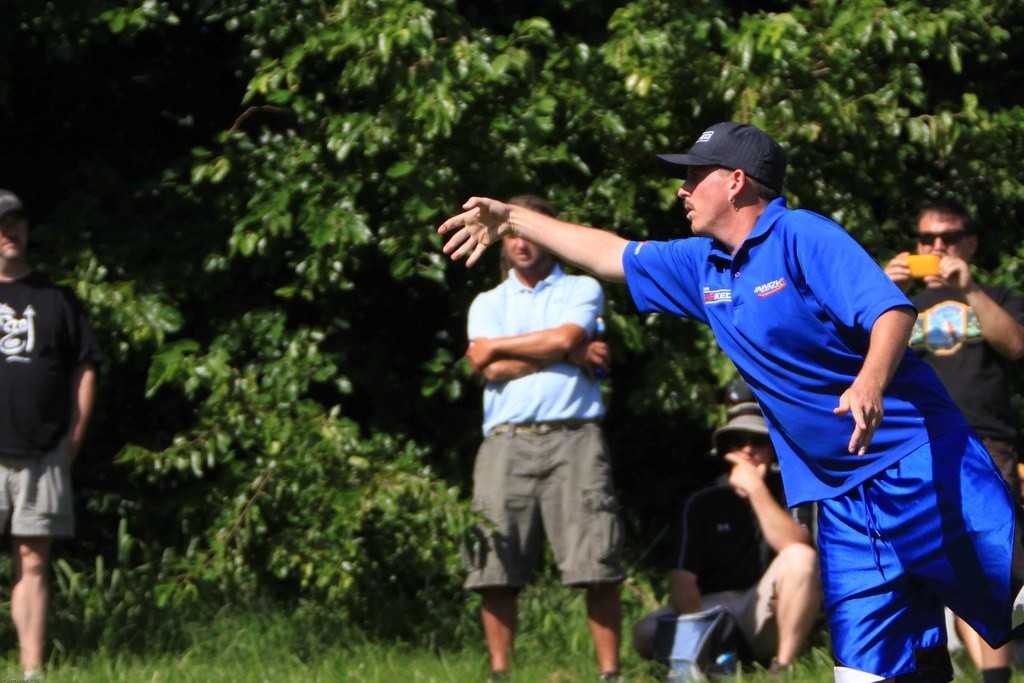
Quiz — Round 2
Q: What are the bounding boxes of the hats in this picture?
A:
[713,402,770,447]
[657,121,785,192]
[0,189,22,219]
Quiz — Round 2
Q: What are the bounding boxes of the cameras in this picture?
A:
[901,255,942,278]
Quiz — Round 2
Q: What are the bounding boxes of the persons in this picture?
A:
[631,402,821,683]
[883,199,1024,683]
[438,121,1024,683]
[463,194,630,683]
[0,191,104,683]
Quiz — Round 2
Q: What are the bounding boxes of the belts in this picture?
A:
[483,423,571,436]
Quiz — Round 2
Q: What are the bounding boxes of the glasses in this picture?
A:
[913,229,969,244]
[725,433,769,449]
